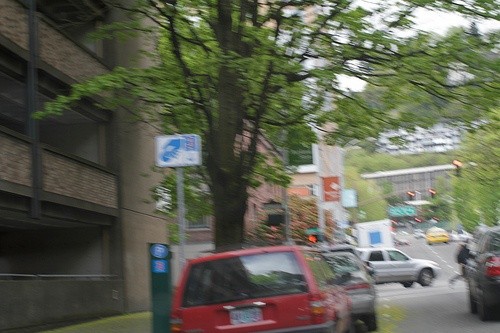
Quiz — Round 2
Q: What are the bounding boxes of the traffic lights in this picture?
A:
[308,235,319,245]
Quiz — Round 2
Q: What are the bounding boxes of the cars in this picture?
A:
[426,227,450,245]
[462,226,500,322]
[321,251,378,333]
[168,246,354,333]
[358,248,440,289]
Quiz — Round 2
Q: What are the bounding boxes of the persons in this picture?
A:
[449,238,476,284]
